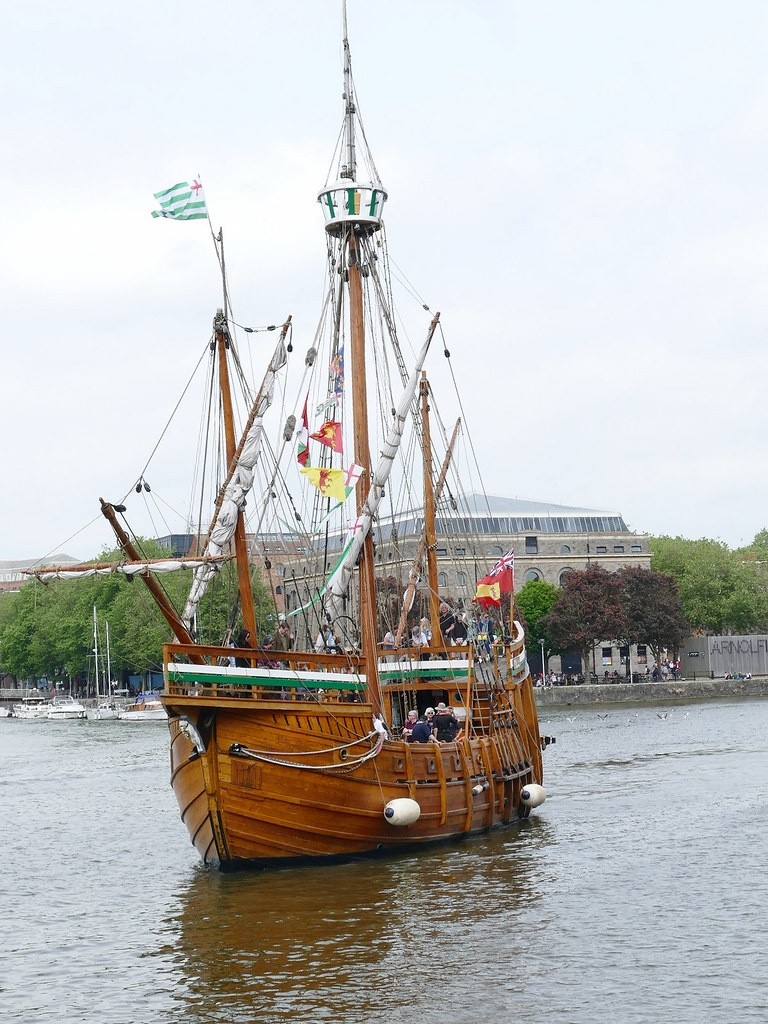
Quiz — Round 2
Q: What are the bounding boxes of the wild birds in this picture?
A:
[566,718,576,723]
[627,708,744,722]
[597,714,608,721]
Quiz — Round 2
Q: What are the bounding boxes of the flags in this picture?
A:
[151,178,207,221]
[471,547,514,611]
[309,422,343,453]
[300,467,346,502]
[295,390,309,467]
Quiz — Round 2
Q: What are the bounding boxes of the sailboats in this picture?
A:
[98,0,544,875]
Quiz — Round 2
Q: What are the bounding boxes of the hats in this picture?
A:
[435,702,448,710]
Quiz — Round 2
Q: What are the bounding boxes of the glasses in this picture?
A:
[485,616,489,618]
[428,712,433,714]
[246,636,250,639]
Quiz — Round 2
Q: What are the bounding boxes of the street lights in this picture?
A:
[537,636,546,691]
[688,650,701,681]
[87,623,102,662]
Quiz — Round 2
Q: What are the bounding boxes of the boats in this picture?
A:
[93,702,118,721]
[1,705,14,719]
[13,696,48,718]
[48,695,87,721]
[119,700,168,719]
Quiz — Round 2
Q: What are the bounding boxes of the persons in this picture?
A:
[234,629,254,698]
[384,602,495,685]
[401,702,463,748]
[256,635,281,699]
[533,669,619,690]
[644,656,680,681]
[724,671,753,680]
[272,621,292,669]
